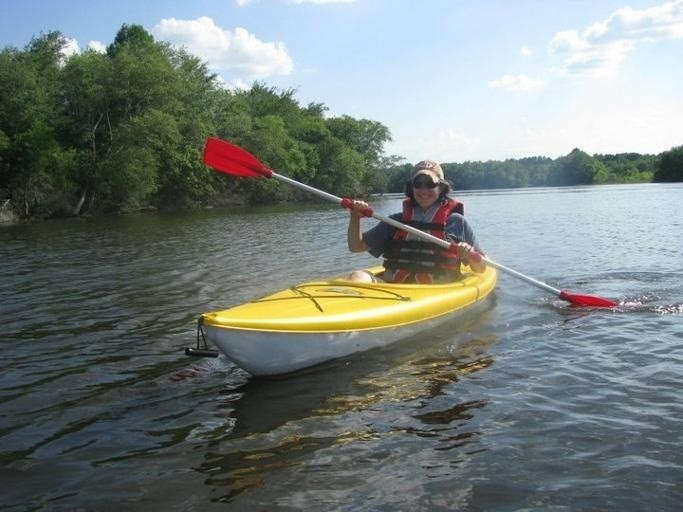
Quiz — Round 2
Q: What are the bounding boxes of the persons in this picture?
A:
[345,161,485,289]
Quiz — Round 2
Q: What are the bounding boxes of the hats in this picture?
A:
[409,160,445,185]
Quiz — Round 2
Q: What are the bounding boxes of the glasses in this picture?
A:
[412,180,439,189]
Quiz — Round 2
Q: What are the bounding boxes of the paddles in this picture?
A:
[203,136,618,308]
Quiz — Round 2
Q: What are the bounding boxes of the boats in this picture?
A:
[197,260,500,379]
